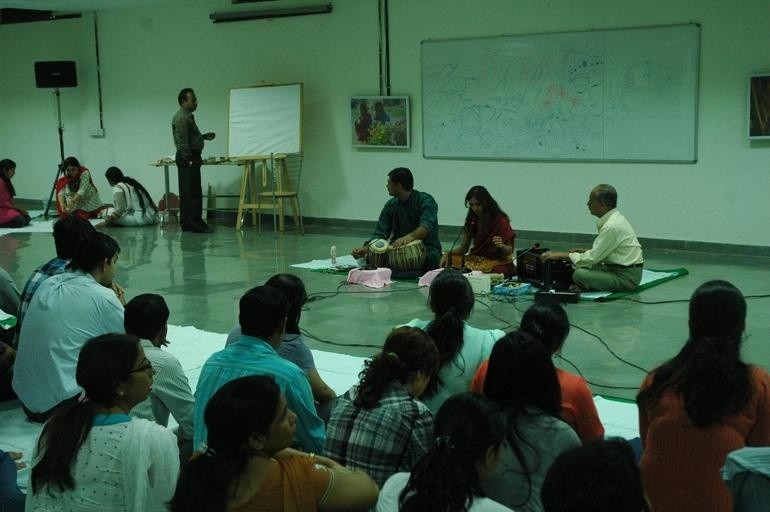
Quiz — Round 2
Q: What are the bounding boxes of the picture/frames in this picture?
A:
[147,155,265,234]
[154,236,249,299]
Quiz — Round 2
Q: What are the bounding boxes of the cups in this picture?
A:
[127,358,152,373]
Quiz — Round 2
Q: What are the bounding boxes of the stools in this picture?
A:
[607,264,643,268]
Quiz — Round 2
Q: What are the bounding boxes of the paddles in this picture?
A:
[1,206,120,233]
[291,242,689,304]
[1,321,643,512]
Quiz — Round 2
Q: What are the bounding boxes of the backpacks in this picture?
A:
[369,238,388,266]
[388,240,426,271]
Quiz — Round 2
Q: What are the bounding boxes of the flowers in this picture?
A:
[458,216,477,273]
[444,216,471,270]
[483,243,540,273]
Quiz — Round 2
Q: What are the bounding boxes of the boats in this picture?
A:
[34,61,77,88]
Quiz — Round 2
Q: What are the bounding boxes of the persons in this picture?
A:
[99,166,160,228]
[373,101,390,123]
[53,156,102,216]
[437,185,516,280]
[1,158,31,229]
[350,166,442,280]
[11,230,127,423]
[1,267,770,511]
[353,102,372,145]
[537,181,644,292]
[10,214,96,380]
[171,90,217,234]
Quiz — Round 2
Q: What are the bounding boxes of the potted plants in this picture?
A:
[746,72,770,143]
[350,95,411,150]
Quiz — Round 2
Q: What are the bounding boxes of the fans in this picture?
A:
[421,22,701,163]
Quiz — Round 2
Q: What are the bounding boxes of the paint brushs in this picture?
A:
[535,289,580,303]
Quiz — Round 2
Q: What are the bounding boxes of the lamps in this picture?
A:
[257,152,306,237]
[236,231,290,290]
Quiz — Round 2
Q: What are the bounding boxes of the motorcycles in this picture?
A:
[44,88,67,217]
